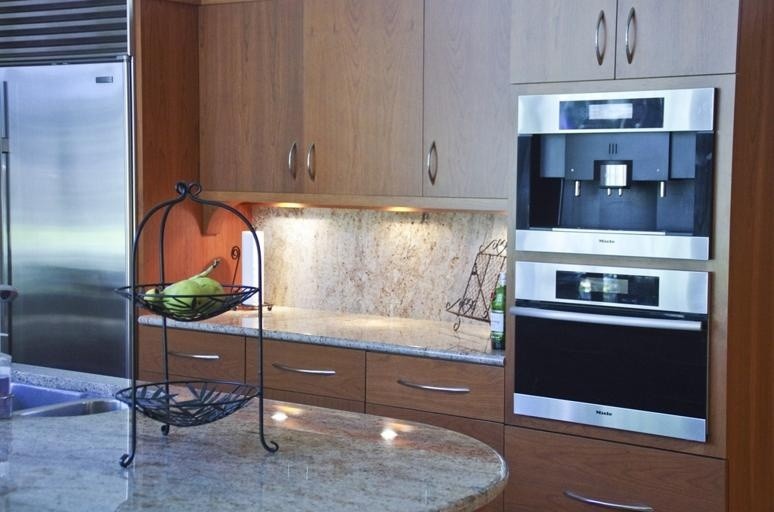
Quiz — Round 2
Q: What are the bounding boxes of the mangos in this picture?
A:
[193,276,224,315]
[162,279,207,320]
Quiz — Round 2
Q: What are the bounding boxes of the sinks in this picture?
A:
[10,379,125,415]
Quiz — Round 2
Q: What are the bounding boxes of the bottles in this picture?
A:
[488,270,506,351]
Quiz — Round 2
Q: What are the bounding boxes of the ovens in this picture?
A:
[509,260,712,443]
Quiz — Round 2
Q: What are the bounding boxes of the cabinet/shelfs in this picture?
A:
[198,3,513,200]
[136,326,365,411]
[363,352,506,456]
[504,0,774,512]
